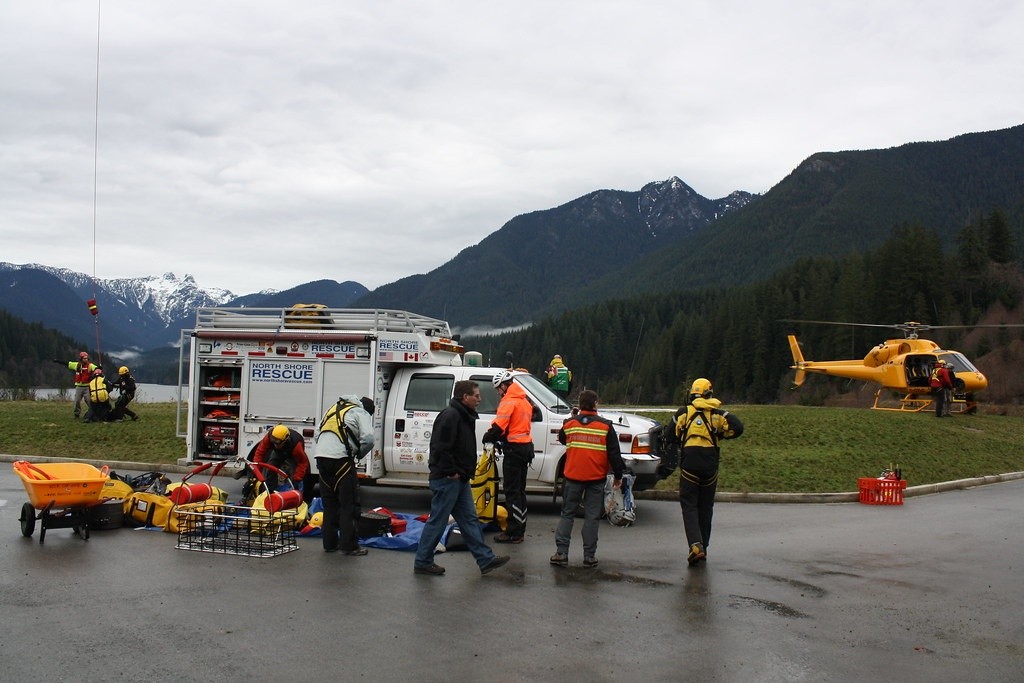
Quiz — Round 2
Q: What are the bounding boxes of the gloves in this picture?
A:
[482,427,500,445]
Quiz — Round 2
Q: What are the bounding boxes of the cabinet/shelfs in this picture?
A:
[199,385,241,424]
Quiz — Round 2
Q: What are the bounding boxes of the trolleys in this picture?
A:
[13,461,113,544]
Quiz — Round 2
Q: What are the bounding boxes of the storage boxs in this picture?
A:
[857,477,907,506]
[392,519,407,534]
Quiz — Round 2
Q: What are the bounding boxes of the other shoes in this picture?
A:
[132,415,138,421]
[947,412,953,416]
[345,548,368,557]
[324,545,340,552]
[943,412,947,416]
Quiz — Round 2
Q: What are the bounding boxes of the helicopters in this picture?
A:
[774,317,1024,413]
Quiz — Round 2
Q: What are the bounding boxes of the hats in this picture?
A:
[362,397,375,415]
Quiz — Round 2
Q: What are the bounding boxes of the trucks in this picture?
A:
[174,302,677,524]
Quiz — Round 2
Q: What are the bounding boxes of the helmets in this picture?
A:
[80,352,88,359]
[492,369,514,389]
[118,366,129,375]
[690,378,713,395]
[938,359,946,365]
[945,363,955,368]
[94,369,102,376]
[271,425,290,445]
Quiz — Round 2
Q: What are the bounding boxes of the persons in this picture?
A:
[313,395,375,557]
[941,362,956,417]
[254,425,308,495]
[666,377,744,566]
[414,379,510,576]
[549,391,624,568]
[543,354,573,400]
[928,359,952,418]
[82,366,139,423]
[482,370,535,544]
[52,351,97,419]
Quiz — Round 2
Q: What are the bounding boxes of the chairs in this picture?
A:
[906,363,932,386]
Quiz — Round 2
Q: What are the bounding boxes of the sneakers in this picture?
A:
[551,553,569,565]
[481,555,510,575]
[494,533,522,544]
[583,556,599,567]
[688,543,705,563]
[414,563,446,576]
